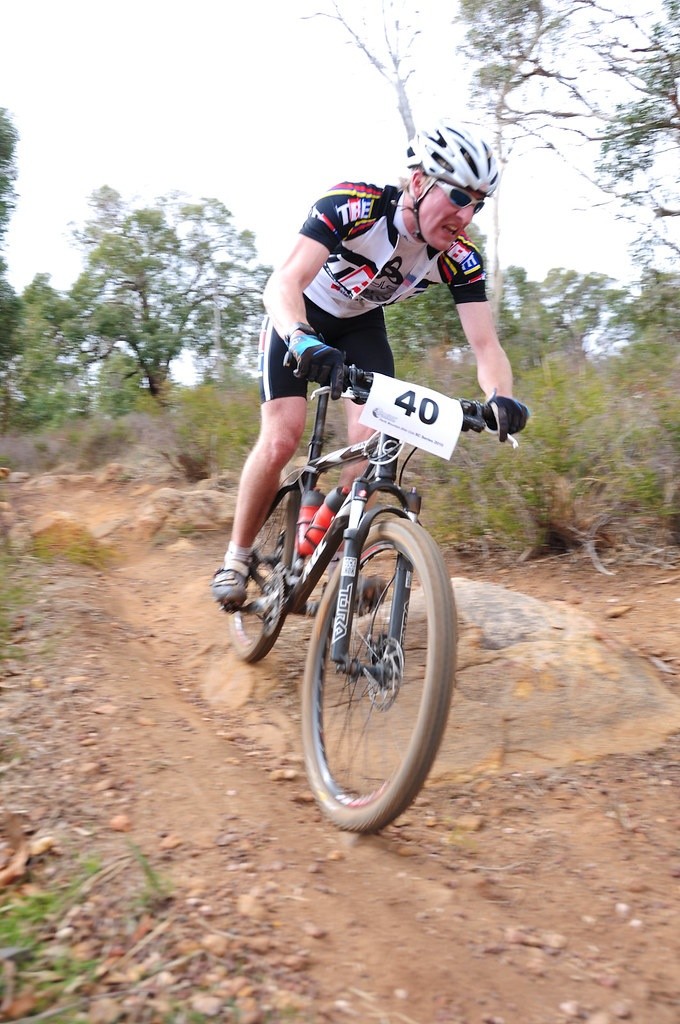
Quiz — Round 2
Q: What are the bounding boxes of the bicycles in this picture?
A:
[226,355,518,832]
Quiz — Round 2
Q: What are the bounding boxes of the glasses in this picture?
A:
[422,170,485,215]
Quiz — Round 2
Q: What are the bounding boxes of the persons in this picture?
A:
[209,125,530,604]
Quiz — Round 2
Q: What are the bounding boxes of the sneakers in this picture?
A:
[211,560,251,606]
[354,574,387,617]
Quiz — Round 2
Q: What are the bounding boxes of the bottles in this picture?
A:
[305,488,350,547]
[296,485,320,558]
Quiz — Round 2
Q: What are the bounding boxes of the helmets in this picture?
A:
[406,125,501,198]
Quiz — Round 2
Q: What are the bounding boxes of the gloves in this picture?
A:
[482,395,528,443]
[288,334,344,401]
